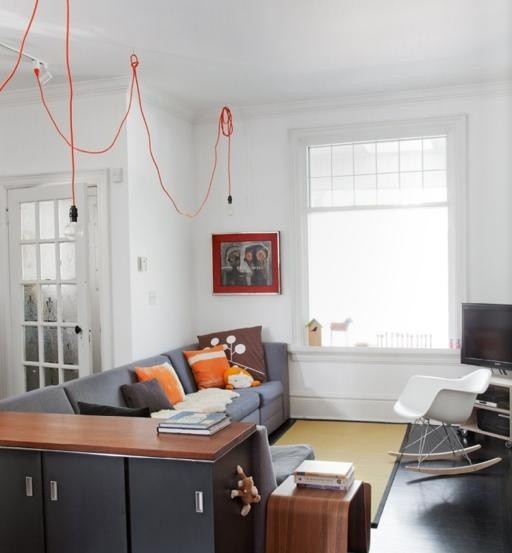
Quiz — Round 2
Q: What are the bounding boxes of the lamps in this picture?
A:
[224,104,236,214]
[63,0,84,243]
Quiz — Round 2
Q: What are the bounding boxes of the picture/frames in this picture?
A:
[210,230,283,295]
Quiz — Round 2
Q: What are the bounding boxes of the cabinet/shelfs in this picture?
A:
[1,444,131,553]
[127,428,263,551]
[456,375,511,446]
[265,473,371,551]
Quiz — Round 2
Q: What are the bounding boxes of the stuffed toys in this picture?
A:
[222,363,261,390]
[232,464,262,515]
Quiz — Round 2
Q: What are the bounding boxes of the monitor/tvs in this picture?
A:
[460,302,512,370]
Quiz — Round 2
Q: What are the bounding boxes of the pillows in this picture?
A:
[133,361,184,404]
[182,346,229,391]
[195,325,268,382]
[77,400,150,417]
[118,377,174,413]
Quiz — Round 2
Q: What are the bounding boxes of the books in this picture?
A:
[157,415,233,436]
[292,458,356,490]
[158,409,228,429]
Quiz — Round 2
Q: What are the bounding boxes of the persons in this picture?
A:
[238,247,254,286]
[254,249,269,285]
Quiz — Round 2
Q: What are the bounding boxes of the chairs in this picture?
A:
[387,367,502,476]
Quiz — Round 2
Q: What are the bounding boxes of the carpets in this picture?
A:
[270,418,410,529]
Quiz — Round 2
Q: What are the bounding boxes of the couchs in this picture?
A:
[1,336,289,442]
[256,422,316,498]
